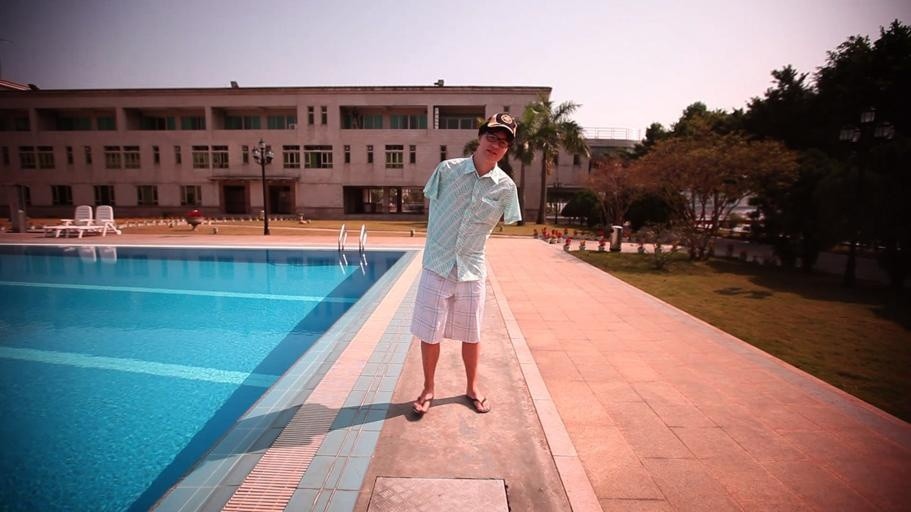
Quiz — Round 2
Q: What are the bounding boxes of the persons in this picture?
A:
[408,113,522,415]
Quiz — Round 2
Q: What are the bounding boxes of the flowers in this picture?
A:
[185,209,206,217]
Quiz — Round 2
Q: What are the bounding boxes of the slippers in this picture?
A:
[466,395,490,413]
[412,396,433,415]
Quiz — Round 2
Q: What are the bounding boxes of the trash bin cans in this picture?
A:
[610,226,623,251]
[17,210,25,233]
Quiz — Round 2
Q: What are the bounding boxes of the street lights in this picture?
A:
[250,136,275,237]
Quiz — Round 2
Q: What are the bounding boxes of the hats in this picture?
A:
[488,112,517,138]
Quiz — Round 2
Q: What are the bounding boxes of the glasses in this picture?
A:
[486,131,511,148]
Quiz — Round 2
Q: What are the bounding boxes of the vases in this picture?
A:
[186,216,205,223]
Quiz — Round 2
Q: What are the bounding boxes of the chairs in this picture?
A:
[42,204,122,239]
